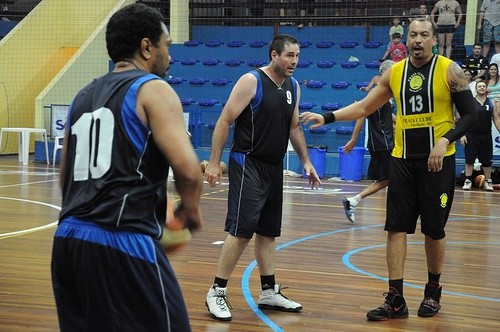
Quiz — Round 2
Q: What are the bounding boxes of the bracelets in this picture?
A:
[321,112,336,125]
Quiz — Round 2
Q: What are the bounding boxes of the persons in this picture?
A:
[298,17,476,322]
[201,36,322,321]
[50,4,202,332]
[341,0,500,222]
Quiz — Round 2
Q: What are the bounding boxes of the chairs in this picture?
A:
[53,135,64,166]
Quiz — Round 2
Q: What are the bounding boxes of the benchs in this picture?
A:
[108,39,409,158]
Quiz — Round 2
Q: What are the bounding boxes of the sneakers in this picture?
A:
[205,284,233,321]
[258,283,302,312]
[417,282,442,317]
[463,179,472,189]
[366,287,409,320]
[483,179,494,191]
[342,197,359,222]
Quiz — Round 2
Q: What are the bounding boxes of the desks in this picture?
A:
[0,128,49,165]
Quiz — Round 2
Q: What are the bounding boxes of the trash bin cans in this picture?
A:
[338,147,367,182]
[302,144,328,179]
